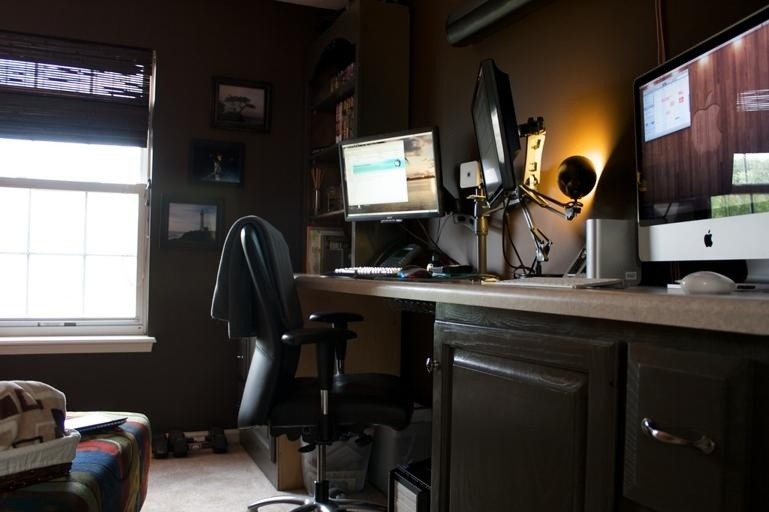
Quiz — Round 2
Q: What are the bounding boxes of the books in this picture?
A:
[306,227,350,275]
[329,62,354,143]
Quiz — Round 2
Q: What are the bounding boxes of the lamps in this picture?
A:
[469,156,597,283]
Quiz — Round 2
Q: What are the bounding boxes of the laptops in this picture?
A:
[64,414,128,434]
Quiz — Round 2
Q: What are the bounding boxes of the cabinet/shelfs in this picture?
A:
[305,62,387,224]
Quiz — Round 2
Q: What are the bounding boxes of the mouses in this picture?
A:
[680,271,737,294]
[398,268,432,278]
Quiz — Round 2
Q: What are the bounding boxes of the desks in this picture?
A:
[236,273,768,511]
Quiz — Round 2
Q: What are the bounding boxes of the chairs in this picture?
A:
[228,221,413,512]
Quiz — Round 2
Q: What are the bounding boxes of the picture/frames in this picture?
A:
[209,76,271,132]
[187,140,246,189]
[159,192,226,255]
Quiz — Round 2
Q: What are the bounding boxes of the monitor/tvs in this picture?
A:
[633,5,769,291]
[338,125,446,222]
[470,58,520,210]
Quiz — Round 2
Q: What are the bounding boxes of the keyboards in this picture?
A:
[496,277,624,289]
[335,266,403,278]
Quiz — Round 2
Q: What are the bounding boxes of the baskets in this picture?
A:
[0,427,81,490]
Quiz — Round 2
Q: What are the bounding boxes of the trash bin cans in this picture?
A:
[300,424,376,496]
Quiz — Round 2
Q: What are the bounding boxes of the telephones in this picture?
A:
[366,240,421,271]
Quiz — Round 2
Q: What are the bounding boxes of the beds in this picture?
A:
[0,409,153,511]
